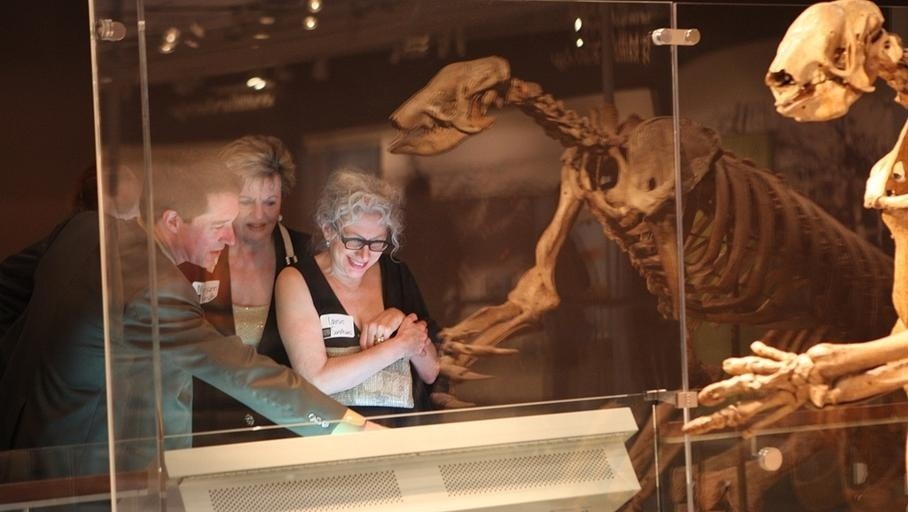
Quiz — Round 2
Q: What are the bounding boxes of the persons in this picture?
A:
[1,153,391,511]
[272,157,443,429]
[2,156,150,386]
[168,131,328,449]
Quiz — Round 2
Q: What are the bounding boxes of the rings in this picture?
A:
[374,334,384,344]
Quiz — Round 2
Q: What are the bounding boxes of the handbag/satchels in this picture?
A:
[317,344,415,409]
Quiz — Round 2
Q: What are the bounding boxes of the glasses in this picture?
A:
[336,229,392,253]
